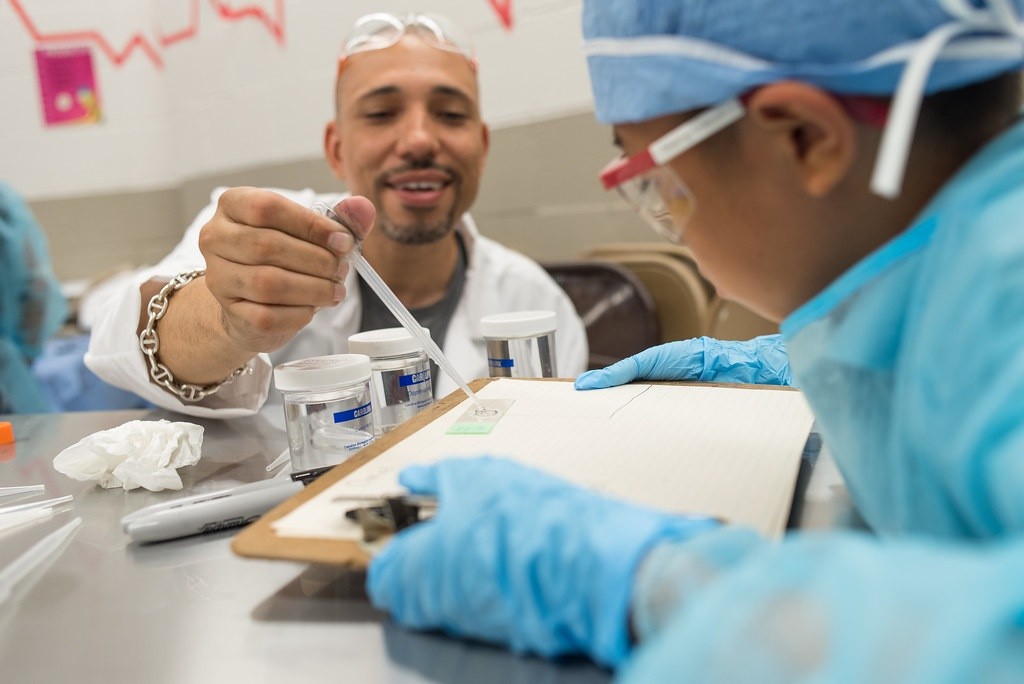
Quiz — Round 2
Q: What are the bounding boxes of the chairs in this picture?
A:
[539,244,780,371]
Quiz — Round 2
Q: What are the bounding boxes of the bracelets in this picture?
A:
[139,266,254,404]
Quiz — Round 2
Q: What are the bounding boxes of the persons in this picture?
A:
[84,13,589,415]
[364,0,1023,684]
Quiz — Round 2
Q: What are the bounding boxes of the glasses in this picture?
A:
[599,93,890,244]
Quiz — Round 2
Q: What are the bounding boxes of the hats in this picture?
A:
[578,0,1022,122]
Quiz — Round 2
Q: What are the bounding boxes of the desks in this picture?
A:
[0,409,613,684]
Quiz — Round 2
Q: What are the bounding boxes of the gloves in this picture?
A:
[573,334,794,392]
[365,454,721,671]
